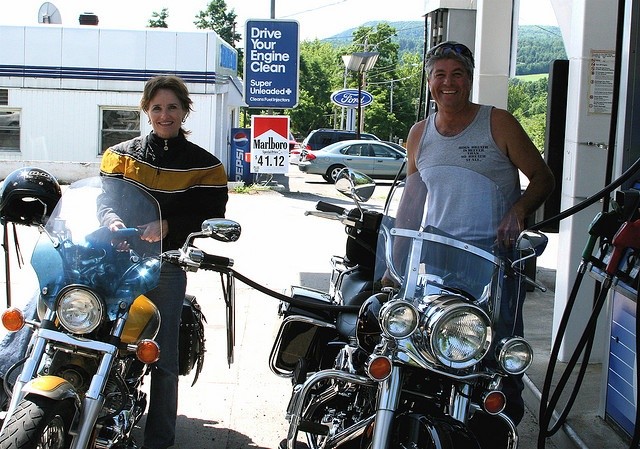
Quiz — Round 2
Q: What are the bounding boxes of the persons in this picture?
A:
[99,74,228,449]
[381,40,556,425]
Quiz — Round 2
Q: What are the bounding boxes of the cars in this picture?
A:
[289,132,301,164]
[298,139,408,183]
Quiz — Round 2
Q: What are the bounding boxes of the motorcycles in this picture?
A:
[0,175,241,449]
[267,167,549,449]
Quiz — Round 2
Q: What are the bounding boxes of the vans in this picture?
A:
[296,129,382,164]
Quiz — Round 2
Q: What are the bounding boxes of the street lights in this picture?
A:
[342,51,381,140]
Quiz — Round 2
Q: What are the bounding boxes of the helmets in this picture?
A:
[0,167,62,232]
